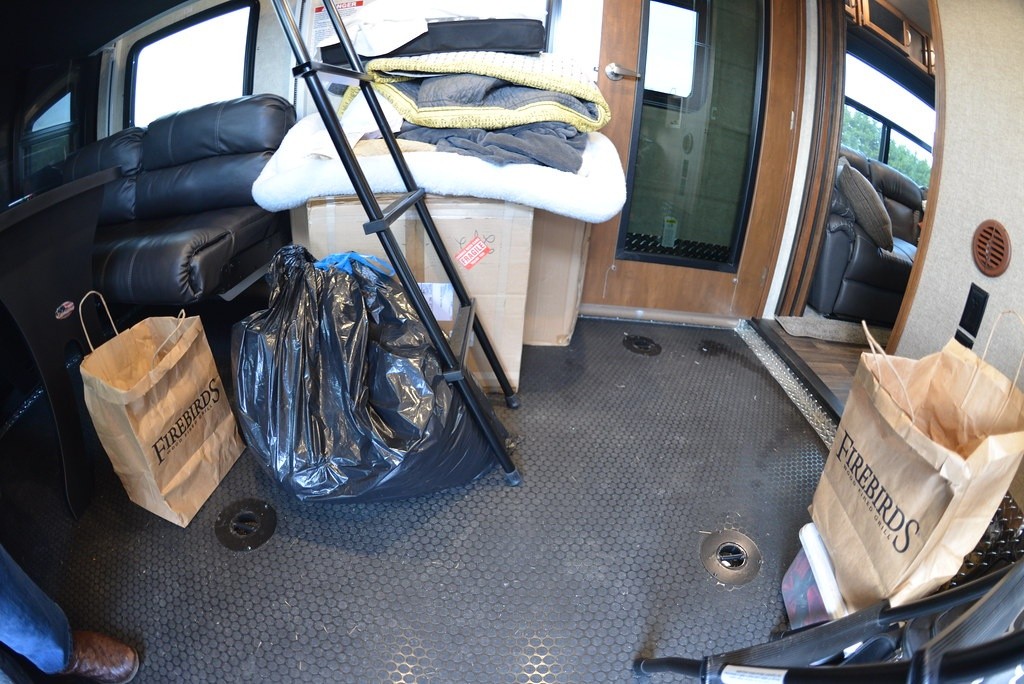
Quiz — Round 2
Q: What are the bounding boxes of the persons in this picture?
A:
[0,543,139,684]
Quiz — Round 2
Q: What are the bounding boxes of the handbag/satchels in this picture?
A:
[79,289,247,530]
[318,19,547,67]
[807,309,1024,621]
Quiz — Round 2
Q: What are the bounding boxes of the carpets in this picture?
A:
[775,305,897,345]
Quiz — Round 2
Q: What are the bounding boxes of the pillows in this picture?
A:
[836,156,893,253]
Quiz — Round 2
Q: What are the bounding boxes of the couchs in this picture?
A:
[808,144,928,328]
[0,93,297,306]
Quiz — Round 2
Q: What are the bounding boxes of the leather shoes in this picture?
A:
[51,626,140,683]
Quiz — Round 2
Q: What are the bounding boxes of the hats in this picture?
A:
[351,12,429,58]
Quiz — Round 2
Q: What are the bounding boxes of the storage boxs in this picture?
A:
[0,164,123,521]
[524,209,591,347]
[307,195,534,393]
[781,522,863,666]
